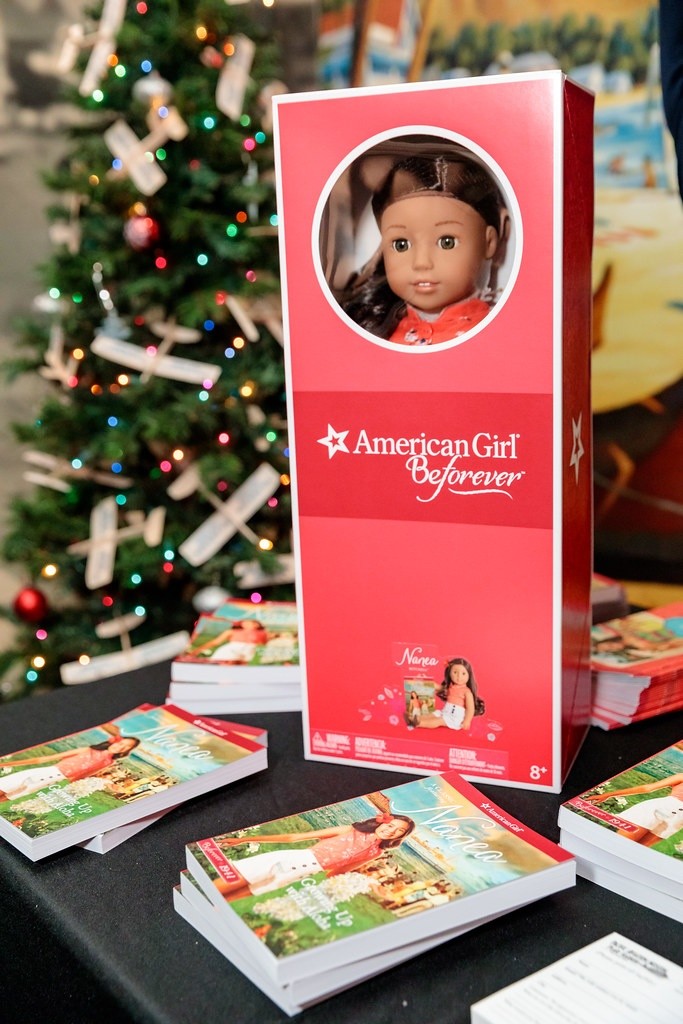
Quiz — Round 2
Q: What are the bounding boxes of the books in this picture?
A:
[593,574,627,626]
[172,769,575,1016]
[470,932,683,1024]
[590,600,683,731]
[165,598,302,716]
[0,703,268,862]
[558,740,683,923]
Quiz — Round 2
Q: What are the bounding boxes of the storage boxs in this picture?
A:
[269,70,593,794]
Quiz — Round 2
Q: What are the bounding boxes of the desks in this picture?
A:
[1,603,680,1024]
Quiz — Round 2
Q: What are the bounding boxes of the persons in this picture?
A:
[332,153,502,347]
[405,657,485,730]
[0,736,141,803]
[214,812,415,902]
[585,772,683,847]
[410,691,422,722]
[187,618,269,664]
